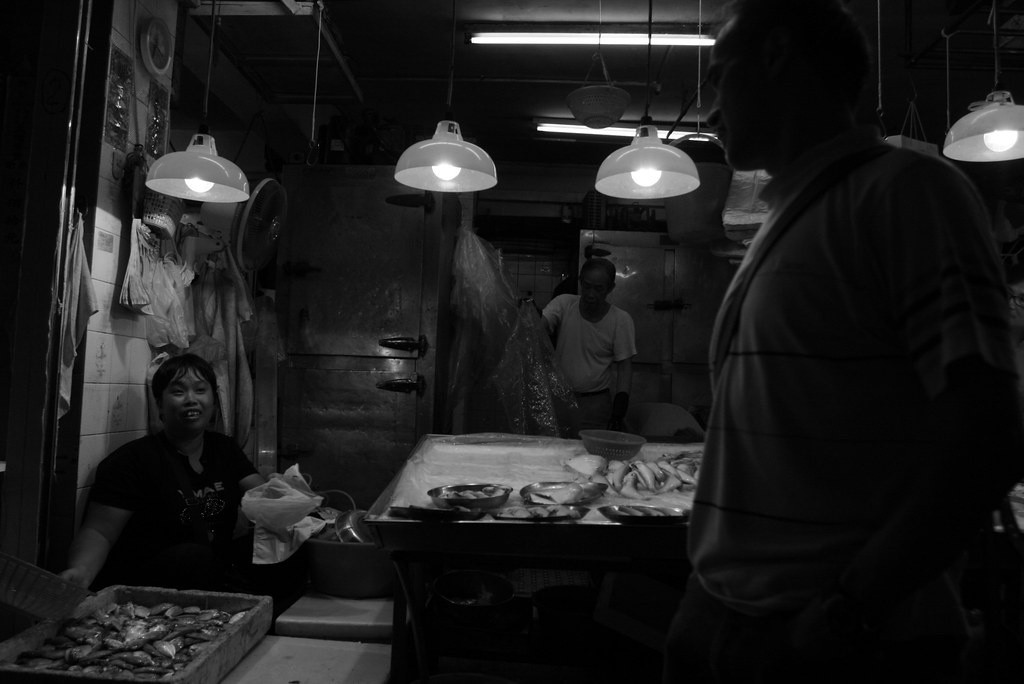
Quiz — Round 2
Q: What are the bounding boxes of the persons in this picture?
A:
[660,0,1024,684]
[55,353,268,591]
[540,258,638,441]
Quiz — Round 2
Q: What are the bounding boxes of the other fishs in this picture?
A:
[441,452,701,517]
[18,601,249,676]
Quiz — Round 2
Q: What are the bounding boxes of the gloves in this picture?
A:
[607,392,629,432]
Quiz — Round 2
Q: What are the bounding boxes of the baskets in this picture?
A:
[142,189,184,240]
[0,552,87,623]
[578,429,647,461]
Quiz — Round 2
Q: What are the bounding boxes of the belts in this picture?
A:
[576,388,609,398]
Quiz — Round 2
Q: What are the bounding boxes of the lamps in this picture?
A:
[532,118,719,143]
[467,28,716,46]
[393,0,497,191]
[942,0,1024,162]
[145,0,250,203]
[595,0,700,200]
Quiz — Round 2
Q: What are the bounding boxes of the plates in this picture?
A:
[427,482,513,509]
[519,481,608,506]
